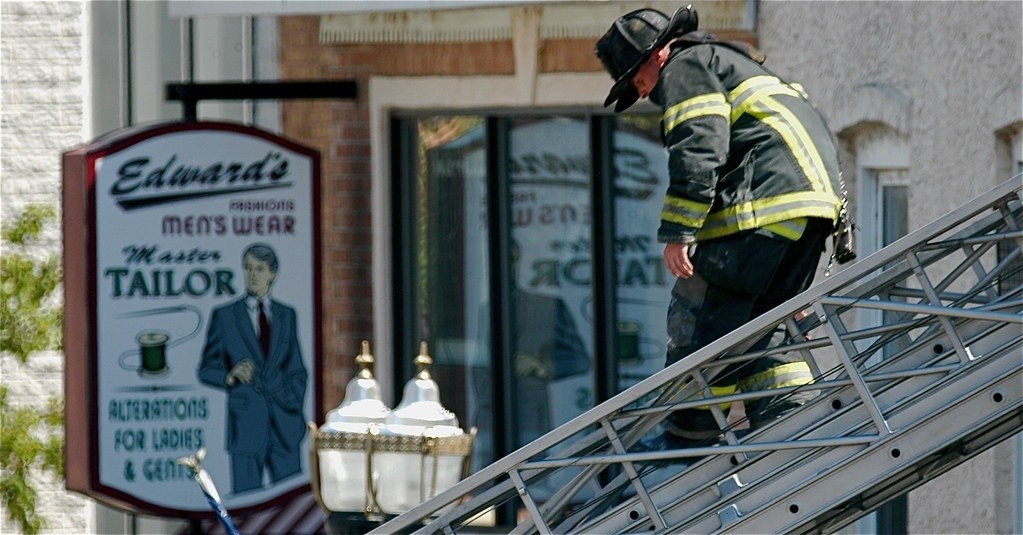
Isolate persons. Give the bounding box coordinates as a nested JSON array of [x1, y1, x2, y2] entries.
[[593, 6, 853, 464]]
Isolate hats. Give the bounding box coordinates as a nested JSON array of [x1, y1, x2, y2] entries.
[[593, 3, 699, 113]]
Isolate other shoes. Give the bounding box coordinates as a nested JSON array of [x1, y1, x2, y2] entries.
[[626, 432, 720, 468]]
